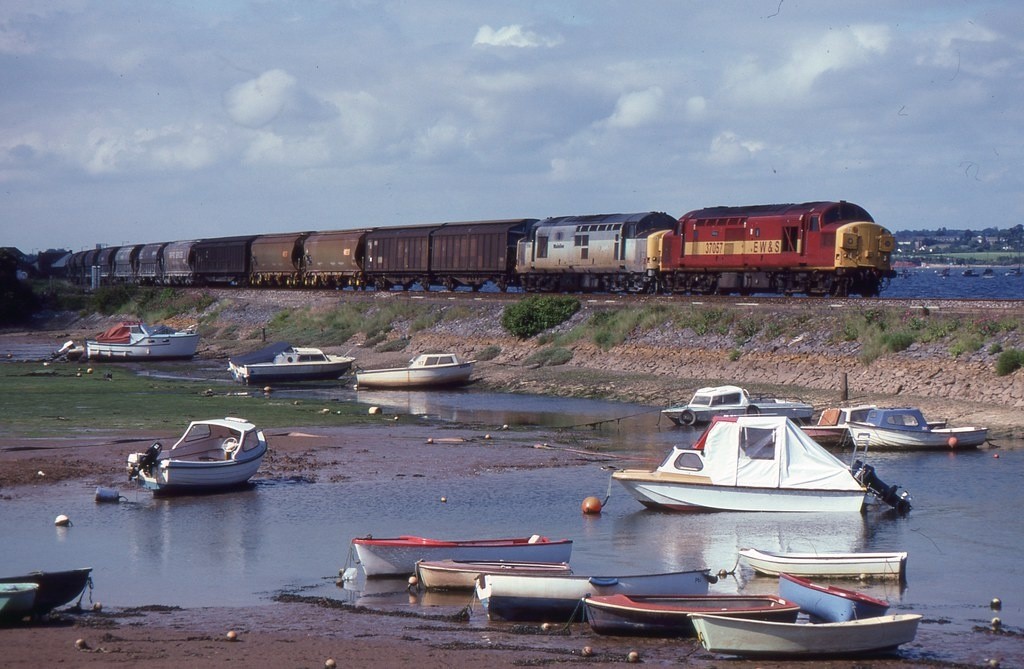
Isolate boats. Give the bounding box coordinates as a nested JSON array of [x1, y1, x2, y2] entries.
[[53, 322, 201, 362], [937, 268, 957, 276], [777, 573, 890, 623], [611, 412, 911, 513], [229, 344, 356, 386], [356, 353, 479, 388], [352, 534, 573, 580], [688, 613, 924, 657], [1005, 268, 1023, 277], [475, 567, 719, 622], [846, 406, 990, 451], [739, 547, 908, 580], [661, 385, 814, 427], [982, 269, 996, 279], [125, 417, 270, 498], [415, 559, 572, 595], [961, 269, 980, 277], [797, 400, 948, 446], [580, 593, 801, 637]]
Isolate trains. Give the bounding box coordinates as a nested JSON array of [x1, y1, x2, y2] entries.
[[68, 200, 899, 299]]
